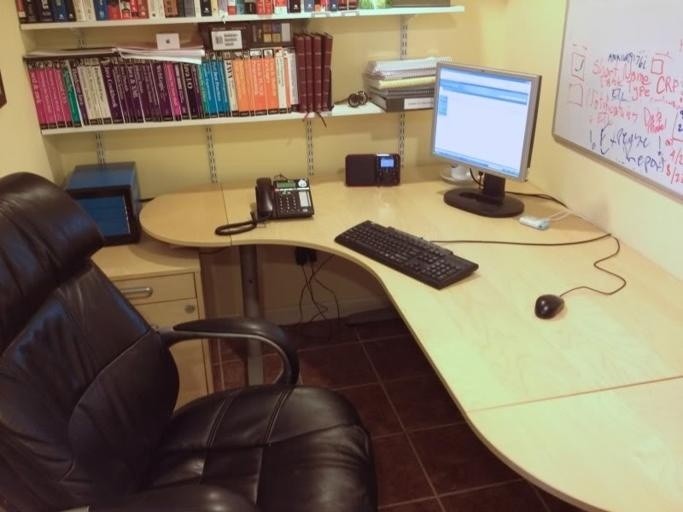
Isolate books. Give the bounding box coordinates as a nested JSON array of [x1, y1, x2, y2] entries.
[[23, 20, 334, 133], [365, 56, 451, 96], [16, 1, 458, 24]]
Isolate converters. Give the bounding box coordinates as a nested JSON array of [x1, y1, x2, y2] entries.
[[519, 216, 550, 230]]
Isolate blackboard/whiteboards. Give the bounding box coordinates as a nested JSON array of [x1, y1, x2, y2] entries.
[[552, 0, 683, 204]]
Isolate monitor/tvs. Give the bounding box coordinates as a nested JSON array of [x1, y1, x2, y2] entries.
[[429, 61, 542, 219]]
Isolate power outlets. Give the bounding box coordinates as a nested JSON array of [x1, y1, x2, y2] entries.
[[297, 247, 317, 266]]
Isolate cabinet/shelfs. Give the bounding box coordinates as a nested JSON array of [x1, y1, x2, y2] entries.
[[88, 241, 215, 412], [20, 4, 466, 136]]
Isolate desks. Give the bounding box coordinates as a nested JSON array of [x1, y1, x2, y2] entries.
[[140, 162, 683, 512]]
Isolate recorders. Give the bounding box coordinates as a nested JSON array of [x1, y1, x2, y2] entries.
[[345, 152, 401, 187]]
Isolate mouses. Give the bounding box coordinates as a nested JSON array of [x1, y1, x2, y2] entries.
[[534, 293, 565, 318]]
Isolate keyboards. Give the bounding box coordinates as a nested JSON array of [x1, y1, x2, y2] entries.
[[336, 219, 478, 290]]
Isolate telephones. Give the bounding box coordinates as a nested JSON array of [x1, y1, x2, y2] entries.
[[253, 177, 315, 224]]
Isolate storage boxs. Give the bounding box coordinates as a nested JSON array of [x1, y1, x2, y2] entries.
[[64, 161, 142, 244]]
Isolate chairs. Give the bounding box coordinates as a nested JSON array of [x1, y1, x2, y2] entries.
[[0, 173, 378, 512]]
